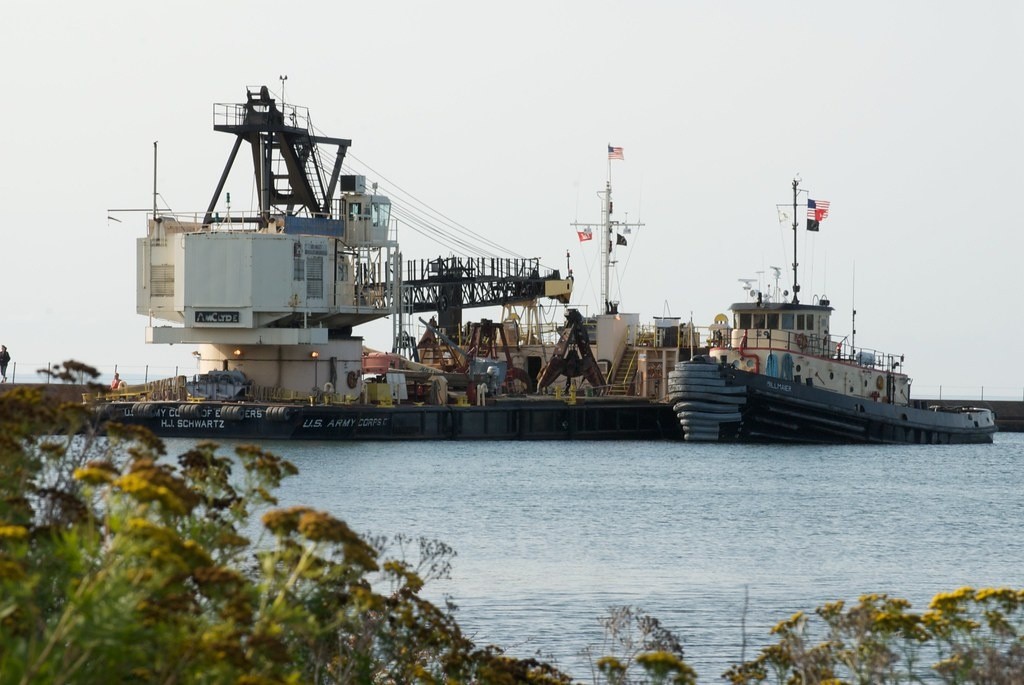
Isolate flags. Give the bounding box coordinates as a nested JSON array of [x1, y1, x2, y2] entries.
[[616, 233, 627, 246], [578, 231, 592, 242], [807, 198, 830, 231], [608, 145, 624, 161]]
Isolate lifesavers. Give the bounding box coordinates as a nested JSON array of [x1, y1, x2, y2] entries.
[[347, 370, 359, 390], [876, 374, 884, 391], [796, 332, 809, 349]]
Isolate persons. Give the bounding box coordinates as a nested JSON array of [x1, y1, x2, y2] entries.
[[0, 345, 11, 383], [110, 373, 124, 389]]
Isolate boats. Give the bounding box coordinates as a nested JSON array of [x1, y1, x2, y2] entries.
[[664, 172, 999, 444], [74, 87, 682, 442]]
